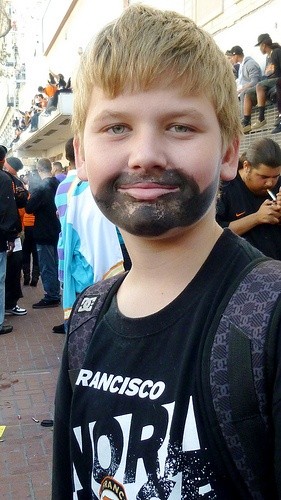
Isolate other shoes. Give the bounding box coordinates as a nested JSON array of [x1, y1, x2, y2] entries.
[[24, 275, 31, 286], [30, 277, 38, 286]]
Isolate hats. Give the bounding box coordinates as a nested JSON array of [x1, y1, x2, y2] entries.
[[254, 34, 269, 47], [5, 157, 23, 174], [227, 45, 243, 55]]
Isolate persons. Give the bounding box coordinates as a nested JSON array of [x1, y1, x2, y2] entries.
[[223, 45, 263, 101], [0, 144, 16, 334], [240, 33, 281, 134], [52, 4, 281, 500], [217, 136, 281, 260], [11, 157, 61, 309], [7, 72, 73, 150], [3, 157, 28, 316], [53, 138, 77, 335]]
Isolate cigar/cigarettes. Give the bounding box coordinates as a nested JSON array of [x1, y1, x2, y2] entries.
[[266, 188, 276, 201]]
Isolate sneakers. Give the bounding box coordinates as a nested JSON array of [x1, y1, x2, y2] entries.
[[251, 119, 267, 129], [52, 323, 65, 334], [270, 123, 281, 134], [273, 114, 281, 126], [5, 304, 27, 316], [32, 299, 59, 309], [0, 325, 13, 334], [242, 124, 252, 133]]
